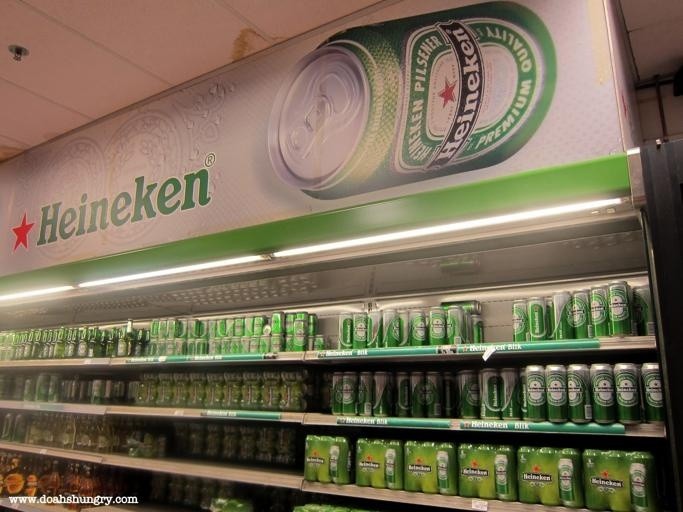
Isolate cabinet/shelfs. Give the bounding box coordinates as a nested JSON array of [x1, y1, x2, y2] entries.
[[0, 330, 679, 511]]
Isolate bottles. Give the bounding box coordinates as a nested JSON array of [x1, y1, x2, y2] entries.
[[23, 409, 170, 456], [0, 317, 136, 359], [0, 450, 116, 510]]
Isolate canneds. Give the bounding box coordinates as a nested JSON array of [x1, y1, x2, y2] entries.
[[266, 0, 558, 198], [438, 256, 479, 274], [0, 277, 666, 512], [564, 231, 637, 250]]
